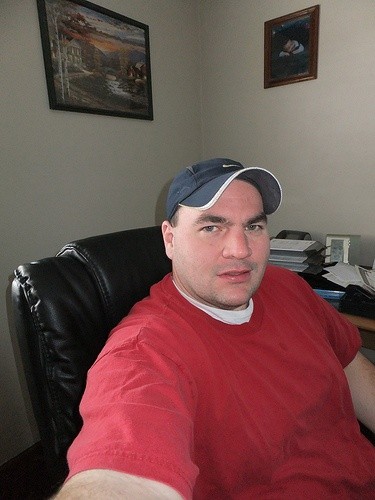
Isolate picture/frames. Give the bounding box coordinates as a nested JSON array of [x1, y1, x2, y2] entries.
[[36, 0, 154, 123], [263, 4, 320, 89]]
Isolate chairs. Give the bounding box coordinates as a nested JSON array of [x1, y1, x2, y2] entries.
[[10, 225, 173, 497]]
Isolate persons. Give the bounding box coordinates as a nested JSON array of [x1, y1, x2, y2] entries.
[[55, 157, 375, 500]]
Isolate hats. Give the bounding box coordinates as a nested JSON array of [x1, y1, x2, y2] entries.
[[164, 158, 282, 218]]
